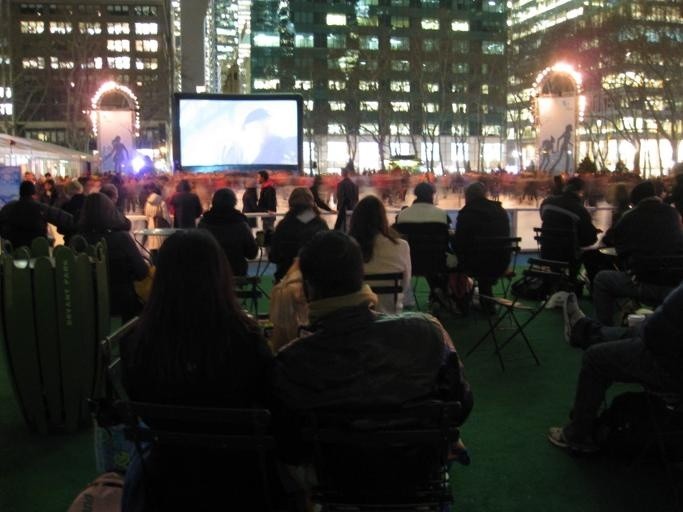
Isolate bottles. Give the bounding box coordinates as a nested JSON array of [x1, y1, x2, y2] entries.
[[257, 311, 272, 353]]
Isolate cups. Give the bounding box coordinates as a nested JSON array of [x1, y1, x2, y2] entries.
[[626, 314, 646, 329]]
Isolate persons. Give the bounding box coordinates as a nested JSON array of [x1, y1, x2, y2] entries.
[[546, 281, 681, 445], [537, 136, 554, 171], [1, 164, 682, 323], [137, 154, 158, 180], [103, 134, 128, 171], [274, 228, 474, 512], [94, 229, 275, 512], [551, 126, 571, 169]]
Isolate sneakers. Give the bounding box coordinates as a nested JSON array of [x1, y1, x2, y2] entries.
[[546, 424, 595, 454], [562, 292, 587, 344]]
